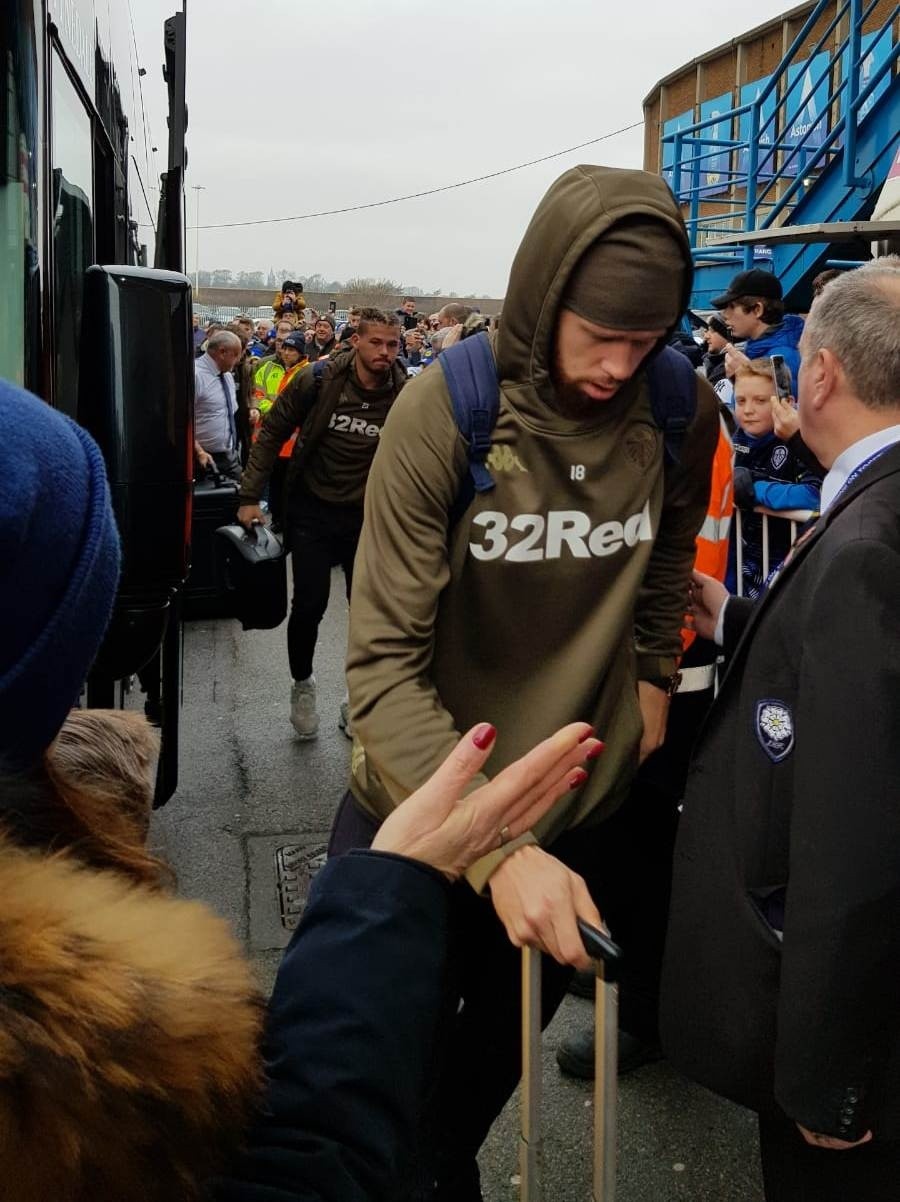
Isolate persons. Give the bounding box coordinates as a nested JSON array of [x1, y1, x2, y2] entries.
[[190, 296, 507, 533], [554, 270, 844, 1081], [272, 281, 306, 323], [192, 330, 242, 481], [330, 163, 722, 1202], [710, 269, 804, 401], [679, 256, 900, 1202], [205, 307, 414, 739], [0, 377, 608, 1202]]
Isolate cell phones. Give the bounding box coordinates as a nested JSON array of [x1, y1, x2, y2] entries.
[[578, 917, 631, 979], [770, 354, 790, 404]]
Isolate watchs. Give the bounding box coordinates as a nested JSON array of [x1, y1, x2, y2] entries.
[[808, 1130, 830, 1143], [642, 670, 684, 697]]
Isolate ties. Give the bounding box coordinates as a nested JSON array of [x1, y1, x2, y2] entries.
[[794, 487, 823, 542], [218, 372, 236, 454]]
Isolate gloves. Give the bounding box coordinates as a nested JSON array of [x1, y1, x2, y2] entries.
[[732, 465, 770, 510]]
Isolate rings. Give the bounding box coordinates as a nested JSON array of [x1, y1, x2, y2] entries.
[[500, 825, 512, 846]]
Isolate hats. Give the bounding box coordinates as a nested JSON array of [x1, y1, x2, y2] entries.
[[710, 311, 734, 340], [0, 379, 121, 798], [711, 269, 782, 310], [281, 330, 306, 354], [562, 216, 688, 329], [314, 314, 335, 332]]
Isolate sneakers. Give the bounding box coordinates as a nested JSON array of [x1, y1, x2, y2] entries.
[[289, 678, 321, 735], [338, 701, 353, 736]]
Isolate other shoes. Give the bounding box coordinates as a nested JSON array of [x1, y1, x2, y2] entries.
[[552, 1025, 664, 1079]]
